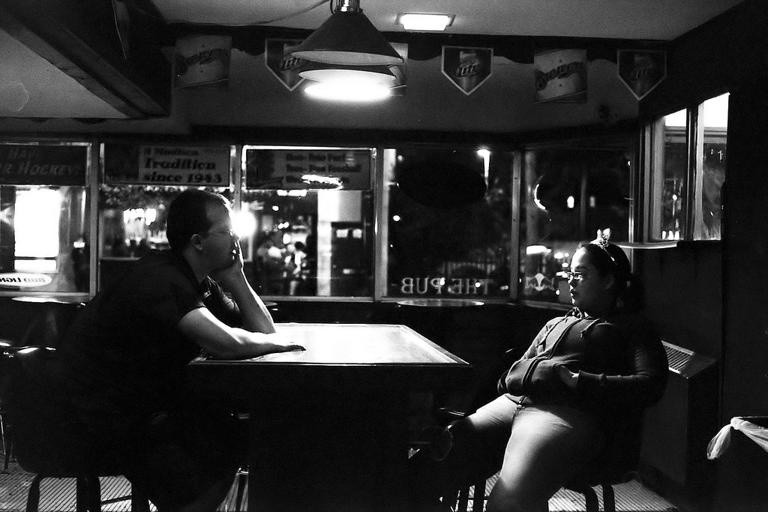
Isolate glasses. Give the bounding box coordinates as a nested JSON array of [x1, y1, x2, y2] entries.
[[198, 226, 235, 235], [563, 271, 608, 280]]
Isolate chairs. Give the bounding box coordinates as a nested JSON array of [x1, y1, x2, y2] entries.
[[7, 346, 148, 510]]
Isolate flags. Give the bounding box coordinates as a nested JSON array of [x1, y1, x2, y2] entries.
[[532, 46, 588, 107], [172, 32, 233, 93], [384, 41, 410, 99]]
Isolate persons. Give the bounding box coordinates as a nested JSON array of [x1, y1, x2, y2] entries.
[[384, 228, 670, 511], [60, 188, 306, 511]]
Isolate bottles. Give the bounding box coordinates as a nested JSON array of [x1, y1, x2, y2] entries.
[[439, 249, 507, 276]]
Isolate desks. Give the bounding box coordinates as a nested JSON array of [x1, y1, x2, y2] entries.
[[189, 322, 473, 510]]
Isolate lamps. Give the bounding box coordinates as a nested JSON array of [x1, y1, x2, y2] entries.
[[292, 4, 403, 106]]
